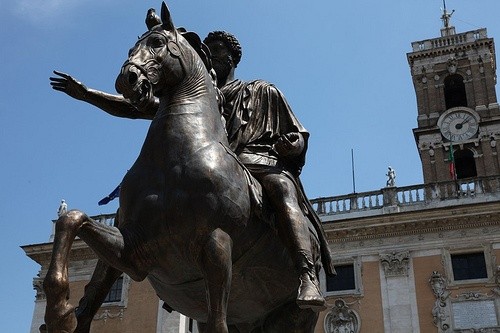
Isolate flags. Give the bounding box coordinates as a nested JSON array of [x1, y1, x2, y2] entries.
[[449, 133, 457, 180]]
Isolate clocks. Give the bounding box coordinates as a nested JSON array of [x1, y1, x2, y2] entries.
[[441, 112, 479, 142]]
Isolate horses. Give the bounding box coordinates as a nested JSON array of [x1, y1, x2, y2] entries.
[[38, 0, 322, 332]]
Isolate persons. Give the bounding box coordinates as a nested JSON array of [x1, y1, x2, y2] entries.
[[49, 31, 330, 313], [57, 199, 68, 217], [385, 166, 396, 187], [440, 9, 455, 28]]
